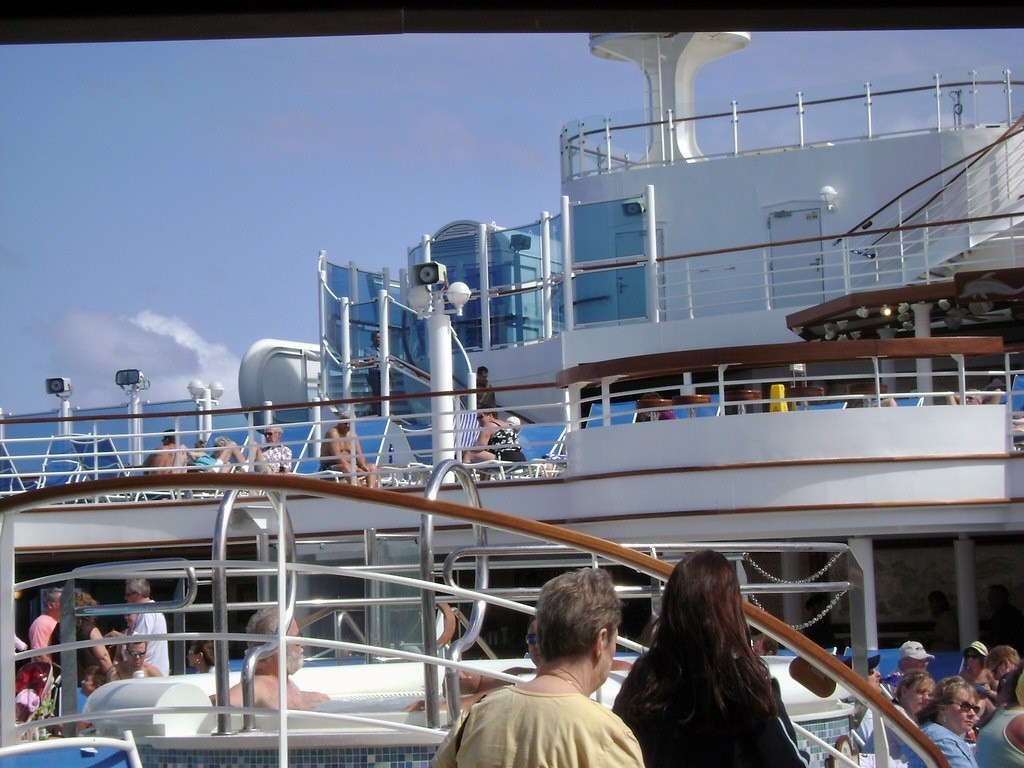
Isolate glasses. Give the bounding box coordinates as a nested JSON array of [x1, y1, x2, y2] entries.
[[949, 702, 981, 713], [963, 652, 981, 661], [526, 634, 538, 644], [476, 413, 487, 421], [868, 668, 877, 676], [263, 431, 277, 437], [127, 649, 147, 657], [991, 662, 1003, 677], [289, 632, 303, 646]]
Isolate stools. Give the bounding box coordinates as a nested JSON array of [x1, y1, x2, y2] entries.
[[636, 400, 672, 421], [726, 389, 760, 414], [784, 386, 825, 410], [671, 395, 711, 418], [846, 384, 888, 407]]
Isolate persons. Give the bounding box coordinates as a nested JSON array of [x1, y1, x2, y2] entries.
[[457, 403, 528, 480], [753, 632, 780, 657], [610, 549, 813, 768], [839, 384, 898, 409], [841, 640, 1024, 768], [475, 365, 499, 420], [403, 614, 634, 711], [945, 387, 1002, 405], [142, 427, 196, 501], [227, 603, 331, 714], [318, 412, 377, 489], [428, 569, 647, 768], [363, 330, 394, 416], [187, 640, 217, 674], [10, 574, 171, 739], [187, 422, 292, 495], [637, 391, 676, 423]]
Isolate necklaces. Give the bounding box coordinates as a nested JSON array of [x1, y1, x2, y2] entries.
[[541, 672, 583, 692]]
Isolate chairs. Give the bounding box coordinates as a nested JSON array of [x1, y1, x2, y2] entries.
[[0, 375, 1024, 504]]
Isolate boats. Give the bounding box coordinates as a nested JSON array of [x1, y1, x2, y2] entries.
[[0, 30, 1024, 767]]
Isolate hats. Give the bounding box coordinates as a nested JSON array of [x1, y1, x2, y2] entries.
[[968, 640, 987, 655], [505, 417, 521, 431], [898, 640, 934, 662], [845, 652, 880, 675]]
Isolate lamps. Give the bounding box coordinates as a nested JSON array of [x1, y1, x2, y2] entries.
[[903, 321, 914, 331], [838, 335, 848, 341], [938, 299, 950, 310], [880, 305, 891, 316], [850, 331, 861, 339], [856, 306, 869, 318], [824, 323, 834, 340], [836, 319, 848, 328], [899, 302, 909, 313], [821, 184, 839, 211]]
